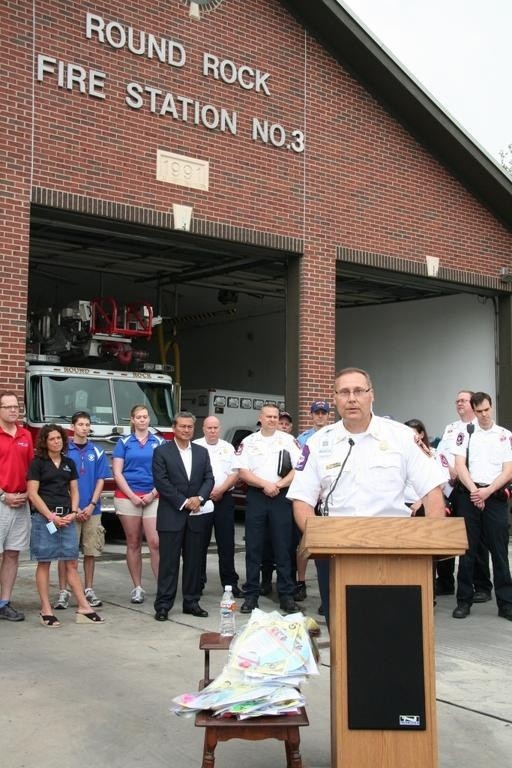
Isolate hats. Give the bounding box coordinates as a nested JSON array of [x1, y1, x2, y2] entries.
[[279, 412, 292, 421], [311, 401, 329, 412]]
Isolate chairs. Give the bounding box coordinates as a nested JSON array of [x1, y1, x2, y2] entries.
[[195, 632, 309, 767]]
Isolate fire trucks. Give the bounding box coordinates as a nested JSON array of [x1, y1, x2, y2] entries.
[[182, 388, 286, 511], [28, 299, 181, 513]]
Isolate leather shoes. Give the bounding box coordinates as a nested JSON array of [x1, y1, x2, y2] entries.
[[156, 609, 168, 621], [241, 598, 259, 614], [453, 607, 470, 619], [498, 608, 512, 621], [183, 606, 208, 617], [434, 586, 454, 594], [224, 586, 245, 598], [472, 592, 491, 602], [200, 591, 202, 595], [280, 600, 300, 613]]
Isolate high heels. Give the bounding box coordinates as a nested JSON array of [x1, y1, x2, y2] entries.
[[40, 613, 61, 628], [76, 612, 104, 624]]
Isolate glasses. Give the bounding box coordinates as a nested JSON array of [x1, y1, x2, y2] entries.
[[0, 406, 19, 408], [335, 388, 371, 396], [455, 399, 470, 403]]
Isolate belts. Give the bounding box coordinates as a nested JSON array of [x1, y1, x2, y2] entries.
[[35, 507, 68, 515]]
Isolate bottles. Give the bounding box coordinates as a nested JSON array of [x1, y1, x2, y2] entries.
[[220, 584, 237, 637]]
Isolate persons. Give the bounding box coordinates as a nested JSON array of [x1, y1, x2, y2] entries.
[[405, 391, 511, 620], [0, 391, 332, 628], [285, 368, 450, 627]]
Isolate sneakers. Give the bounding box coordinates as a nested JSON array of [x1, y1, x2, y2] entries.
[[54, 590, 71, 608], [131, 587, 146, 603], [242, 583, 247, 590], [294, 587, 308, 601], [318, 605, 324, 615], [259, 584, 272, 595], [1, 602, 24, 621], [84, 590, 102, 607]]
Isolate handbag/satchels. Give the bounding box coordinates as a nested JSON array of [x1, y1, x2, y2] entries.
[[448, 480, 480, 520]]
